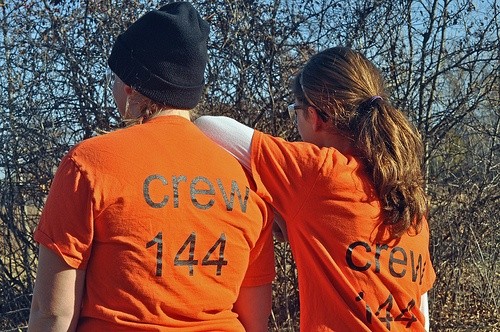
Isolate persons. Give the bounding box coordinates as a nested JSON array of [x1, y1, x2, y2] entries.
[[193, 45, 436, 332], [27, 1, 277, 332]]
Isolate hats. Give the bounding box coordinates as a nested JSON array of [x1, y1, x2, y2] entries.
[[108, 2, 210, 108]]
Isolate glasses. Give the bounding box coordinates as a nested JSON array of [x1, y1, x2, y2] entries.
[[288, 103, 330, 123]]
